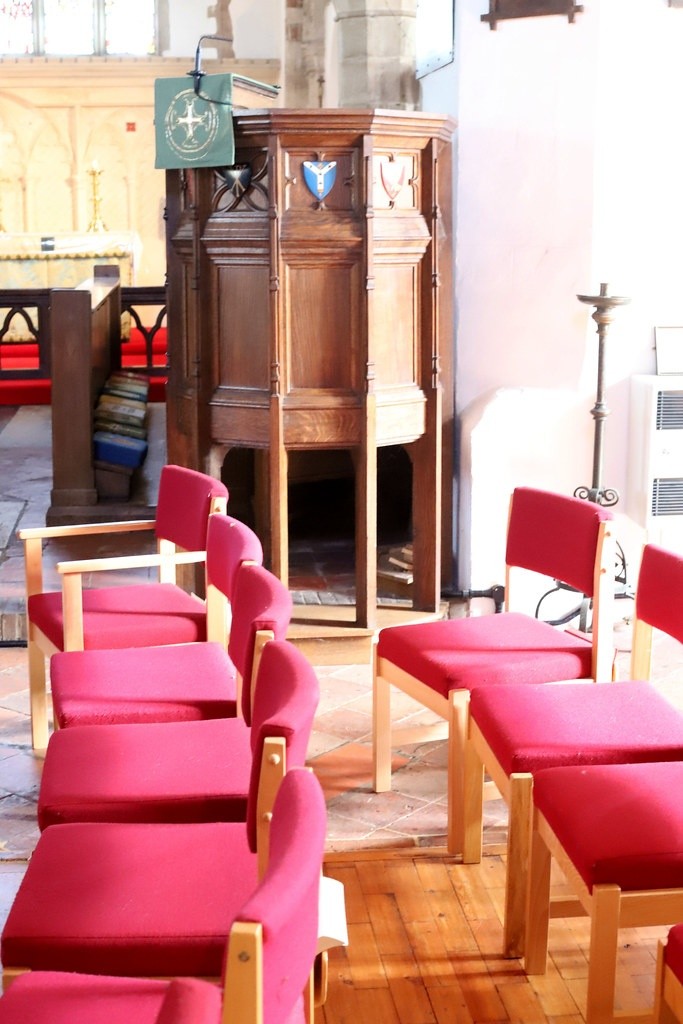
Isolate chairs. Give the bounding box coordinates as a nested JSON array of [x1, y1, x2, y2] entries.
[[0, 465, 683, 1024]]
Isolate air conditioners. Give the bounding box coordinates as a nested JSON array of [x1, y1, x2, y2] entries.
[[626, 371, 683, 560]]
